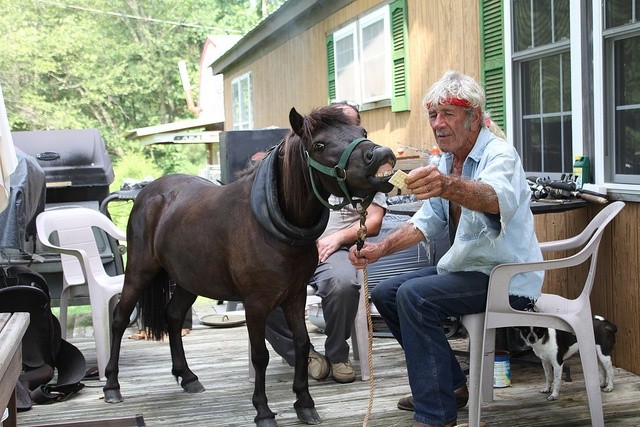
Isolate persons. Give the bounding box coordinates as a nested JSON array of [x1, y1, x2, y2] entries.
[[350, 70, 545, 427], [251, 102, 390, 383]]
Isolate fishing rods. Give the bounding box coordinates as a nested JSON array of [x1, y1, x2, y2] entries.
[[397, 141, 609, 204]]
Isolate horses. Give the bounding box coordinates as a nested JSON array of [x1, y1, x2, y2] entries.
[[104, 103, 396, 427]]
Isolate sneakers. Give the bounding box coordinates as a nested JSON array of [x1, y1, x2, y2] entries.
[[306, 345, 328, 380], [329, 360, 356, 380]]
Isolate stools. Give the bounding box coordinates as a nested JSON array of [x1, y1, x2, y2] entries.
[[0, 310, 31, 422]]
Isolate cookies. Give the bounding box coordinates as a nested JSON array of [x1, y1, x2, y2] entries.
[[388, 170, 409, 191]]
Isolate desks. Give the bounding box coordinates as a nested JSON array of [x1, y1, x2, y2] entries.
[[386, 197, 587, 376]]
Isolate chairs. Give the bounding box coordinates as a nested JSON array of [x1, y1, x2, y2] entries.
[[460, 201, 626, 427], [36, 205, 127, 382], [249, 268, 371, 385]]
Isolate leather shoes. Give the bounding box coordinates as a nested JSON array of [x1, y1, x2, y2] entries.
[[407, 421, 459, 427], [397, 387, 470, 411]]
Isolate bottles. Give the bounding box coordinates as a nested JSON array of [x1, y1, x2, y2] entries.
[[493, 351, 511, 388]]
